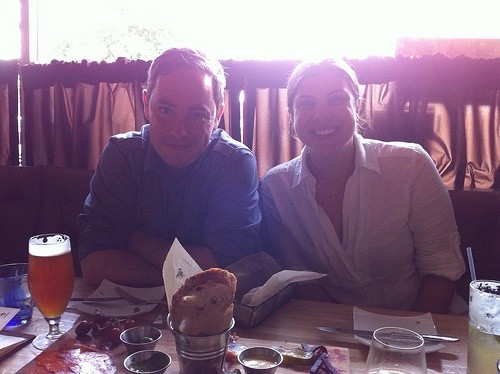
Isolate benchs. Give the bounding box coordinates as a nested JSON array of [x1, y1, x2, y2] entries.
[[0, 167, 500, 315]]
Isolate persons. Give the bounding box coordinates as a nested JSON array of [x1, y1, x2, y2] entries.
[[261, 57, 469, 316], [76, 47, 262, 288]]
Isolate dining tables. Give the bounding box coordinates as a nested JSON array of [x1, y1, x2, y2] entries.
[[0, 278, 470, 374]]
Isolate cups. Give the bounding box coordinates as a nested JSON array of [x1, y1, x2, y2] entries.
[[167, 311, 235, 374], [0, 263, 33, 326], [365, 327, 427, 374], [467, 279, 500, 374]]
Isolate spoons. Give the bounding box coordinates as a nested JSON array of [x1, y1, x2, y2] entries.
[[114, 286, 167, 306]]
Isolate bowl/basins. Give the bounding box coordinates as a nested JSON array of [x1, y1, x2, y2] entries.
[[120, 326, 162, 352], [237, 346, 283, 374], [124, 350, 172, 374]]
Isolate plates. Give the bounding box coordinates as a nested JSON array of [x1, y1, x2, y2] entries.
[[84, 279, 166, 316], [352, 306, 446, 354]]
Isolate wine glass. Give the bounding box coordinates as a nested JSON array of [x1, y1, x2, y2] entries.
[[28, 233, 75, 350]]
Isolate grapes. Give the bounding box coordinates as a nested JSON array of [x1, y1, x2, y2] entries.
[[75, 311, 137, 346]]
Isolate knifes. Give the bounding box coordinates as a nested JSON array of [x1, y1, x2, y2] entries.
[[69, 296, 125, 302], [315, 326, 460, 343]]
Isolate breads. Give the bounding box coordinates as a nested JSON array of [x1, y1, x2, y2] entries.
[[171, 268, 237, 336]]
